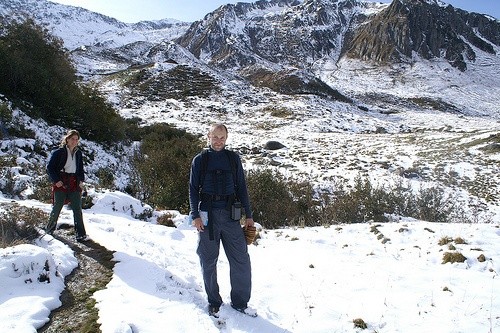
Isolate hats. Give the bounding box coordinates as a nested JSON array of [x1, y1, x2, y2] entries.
[[243, 227, 256, 244]]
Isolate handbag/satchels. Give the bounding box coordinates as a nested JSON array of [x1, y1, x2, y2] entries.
[[232, 201, 242, 220]]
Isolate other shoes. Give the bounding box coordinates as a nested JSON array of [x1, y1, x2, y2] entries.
[[45, 231, 52, 234], [76, 236, 86, 241], [231, 302, 257, 318], [205, 309, 219, 318]]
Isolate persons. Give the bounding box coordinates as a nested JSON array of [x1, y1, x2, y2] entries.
[[188, 123, 259, 329], [45, 130, 89, 242]]
